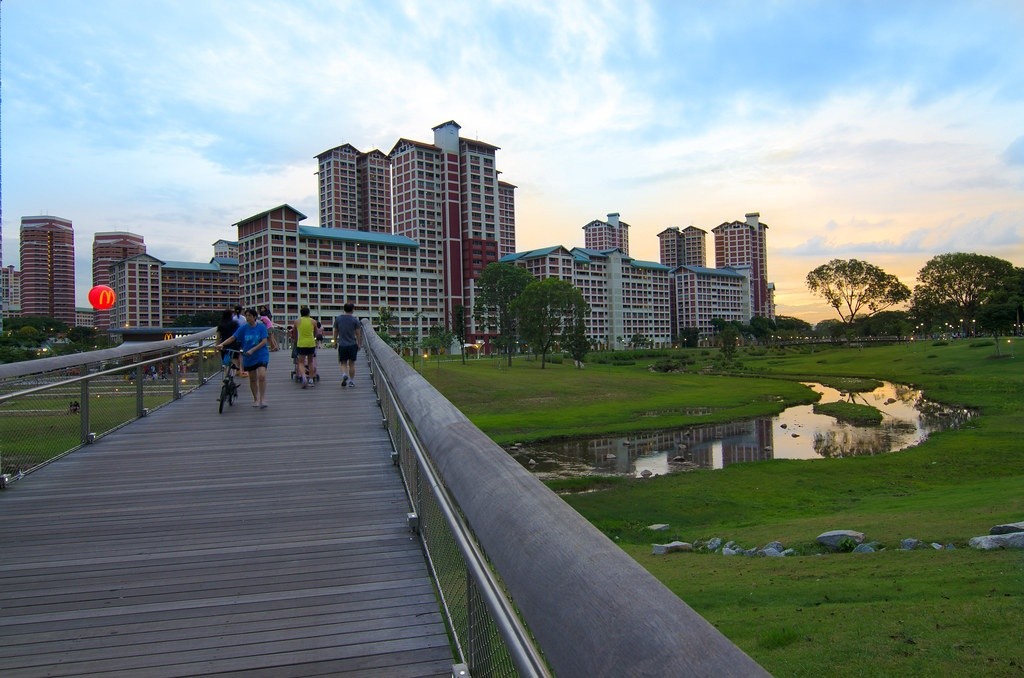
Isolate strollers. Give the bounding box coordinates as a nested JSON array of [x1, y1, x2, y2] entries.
[[291, 341, 320, 382]]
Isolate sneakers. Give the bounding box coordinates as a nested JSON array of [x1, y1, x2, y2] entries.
[[340, 373, 348, 386], [259, 401, 268, 408], [348, 380, 355, 387], [252, 401, 260, 407], [308, 379, 314, 387], [301, 380, 307, 388]]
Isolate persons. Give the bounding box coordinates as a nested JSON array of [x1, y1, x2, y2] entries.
[[214, 303, 325, 375], [215, 309, 272, 408], [73, 401, 80, 410], [127, 362, 190, 381], [949, 329, 977, 339], [332, 302, 362, 388], [291, 307, 320, 389], [69, 401, 75, 410]]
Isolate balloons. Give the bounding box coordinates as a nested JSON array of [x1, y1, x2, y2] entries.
[[88, 285, 116, 309]]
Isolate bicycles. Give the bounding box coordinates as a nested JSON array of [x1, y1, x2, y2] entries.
[[216, 348, 247, 414]]
